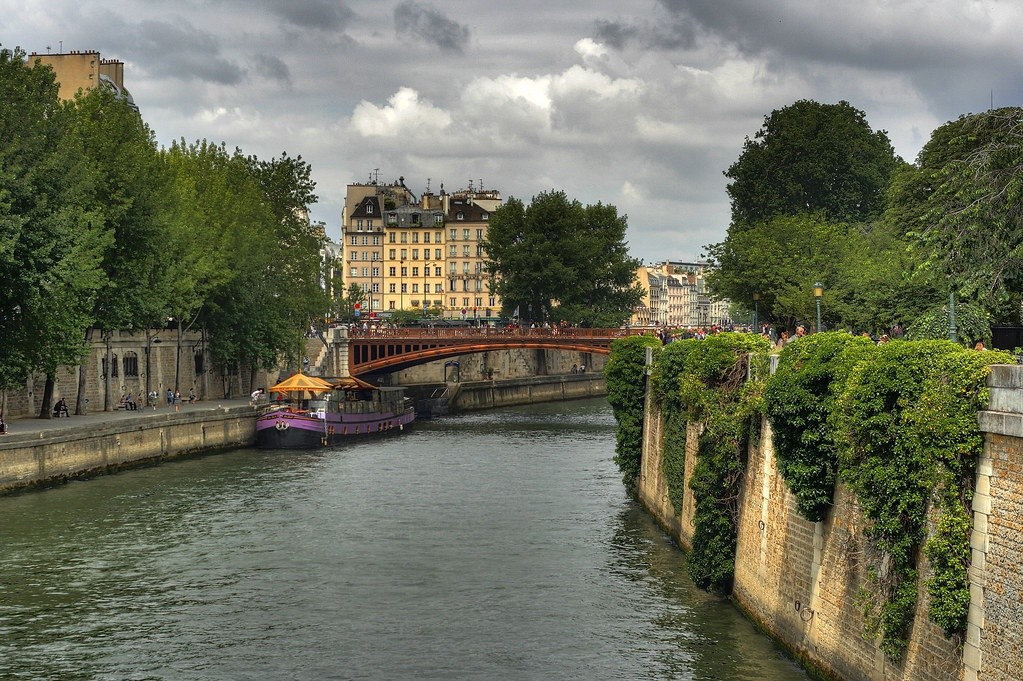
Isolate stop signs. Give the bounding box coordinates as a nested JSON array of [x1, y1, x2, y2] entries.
[[370, 313, 374, 317], [461, 310, 466, 315], [354, 304, 360, 309]]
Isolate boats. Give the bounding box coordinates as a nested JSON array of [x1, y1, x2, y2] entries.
[[254, 370, 416, 449]]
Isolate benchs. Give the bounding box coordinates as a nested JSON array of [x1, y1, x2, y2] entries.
[[52, 410, 66, 417], [117, 402, 131, 410], [180, 397, 190, 403]]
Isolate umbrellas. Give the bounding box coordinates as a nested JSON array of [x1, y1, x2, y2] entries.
[[267, 368, 331, 411]]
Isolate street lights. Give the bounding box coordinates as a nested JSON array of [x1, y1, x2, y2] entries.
[[752, 290, 760, 333], [704, 306, 706, 327], [424, 260, 436, 308], [812, 281, 825, 332], [695, 305, 701, 330], [368, 258, 382, 313], [367, 289, 371, 329], [399, 261, 404, 312]]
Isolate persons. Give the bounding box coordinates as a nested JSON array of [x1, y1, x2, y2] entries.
[[703, 323, 734, 336], [167, 388, 173, 407], [188, 388, 197, 404], [465, 323, 523, 336], [352, 322, 399, 337], [420, 321, 435, 337], [529, 319, 570, 336], [656, 323, 705, 345], [136, 389, 146, 413], [303, 355, 310, 371], [248, 389, 262, 405], [172, 389, 181, 412], [120, 395, 130, 410], [151, 389, 159, 410], [126, 392, 137, 410], [54, 398, 70, 417], [746, 322, 804, 348]]
